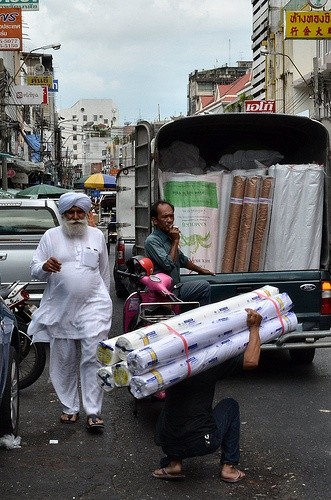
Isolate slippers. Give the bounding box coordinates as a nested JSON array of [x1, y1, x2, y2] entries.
[[221, 467, 247, 482], [61, 411, 80, 423], [86, 414, 104, 428], [153, 467, 186, 478]]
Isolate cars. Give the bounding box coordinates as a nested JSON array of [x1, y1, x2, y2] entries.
[[0, 198, 63, 310], [0, 296, 24, 443]]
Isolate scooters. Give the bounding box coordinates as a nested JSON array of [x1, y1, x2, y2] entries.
[[115, 255, 200, 423]]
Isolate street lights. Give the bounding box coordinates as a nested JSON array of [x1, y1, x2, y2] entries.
[[259, 49, 320, 120], [54, 118, 85, 190], [0, 40, 62, 192]]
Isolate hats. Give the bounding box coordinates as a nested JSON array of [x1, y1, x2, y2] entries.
[[58, 191, 91, 214]]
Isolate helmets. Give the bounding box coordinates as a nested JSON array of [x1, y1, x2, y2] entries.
[[126, 255, 154, 288]]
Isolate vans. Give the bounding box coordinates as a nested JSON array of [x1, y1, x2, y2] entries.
[[114, 111, 330, 372]]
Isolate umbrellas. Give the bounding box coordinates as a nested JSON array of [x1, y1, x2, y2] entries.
[[18, 183, 67, 195], [75, 172, 118, 188]]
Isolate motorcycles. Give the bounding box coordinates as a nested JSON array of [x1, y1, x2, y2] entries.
[[0, 280, 46, 391]]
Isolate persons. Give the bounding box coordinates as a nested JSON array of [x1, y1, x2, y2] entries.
[[150, 309, 264, 481], [27, 193, 113, 428], [144, 200, 216, 305]]
